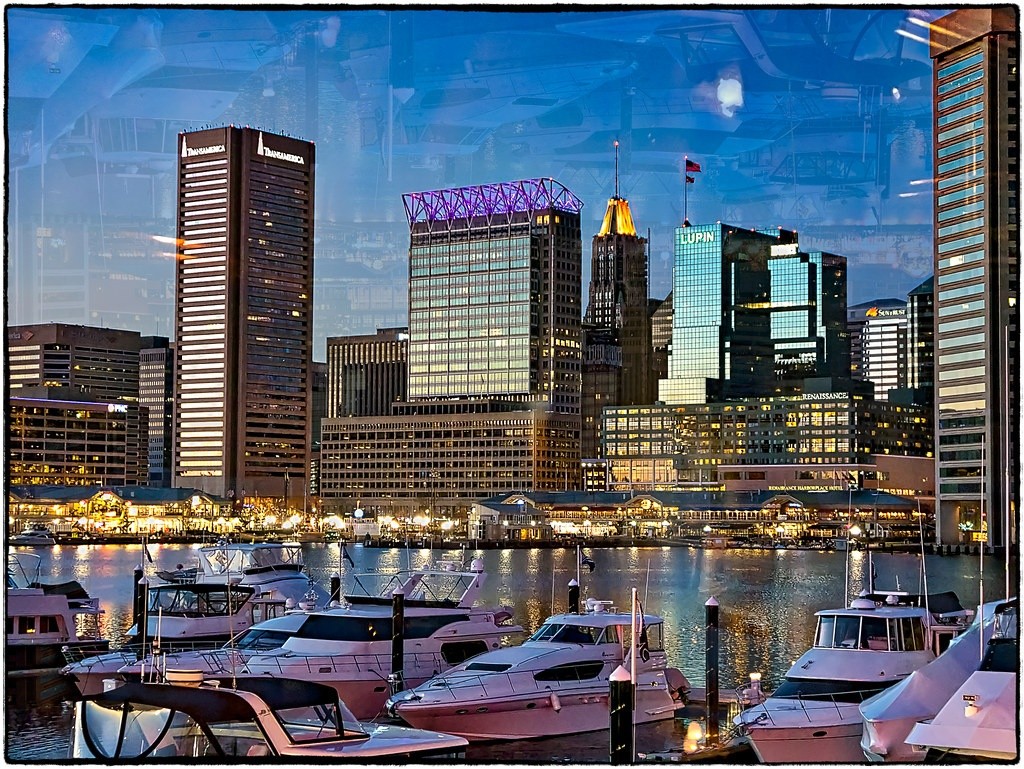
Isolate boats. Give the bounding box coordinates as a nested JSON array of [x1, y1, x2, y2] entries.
[[215, 540, 228, 547], [56, 542, 690, 767], [9, 525, 59, 545], [731, 485, 1016, 764], [6, 553, 111, 678], [739, 542, 826, 550], [282, 542, 302, 548]]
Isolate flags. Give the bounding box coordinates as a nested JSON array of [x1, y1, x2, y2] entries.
[[686, 161, 700, 172], [580, 552, 595, 573], [686, 176, 694, 183], [638, 602, 650, 663], [144, 546, 152, 563], [343, 546, 354, 567]]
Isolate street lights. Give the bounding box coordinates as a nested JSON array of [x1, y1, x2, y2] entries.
[[775, 526, 783, 541], [80, 500, 85, 531], [703, 525, 712, 539], [583, 520, 591, 536], [429, 469, 440, 527], [631, 521, 636, 535], [503, 520, 508, 539], [126, 501, 131, 532], [662, 521, 670, 538], [531, 521, 536, 539]]
